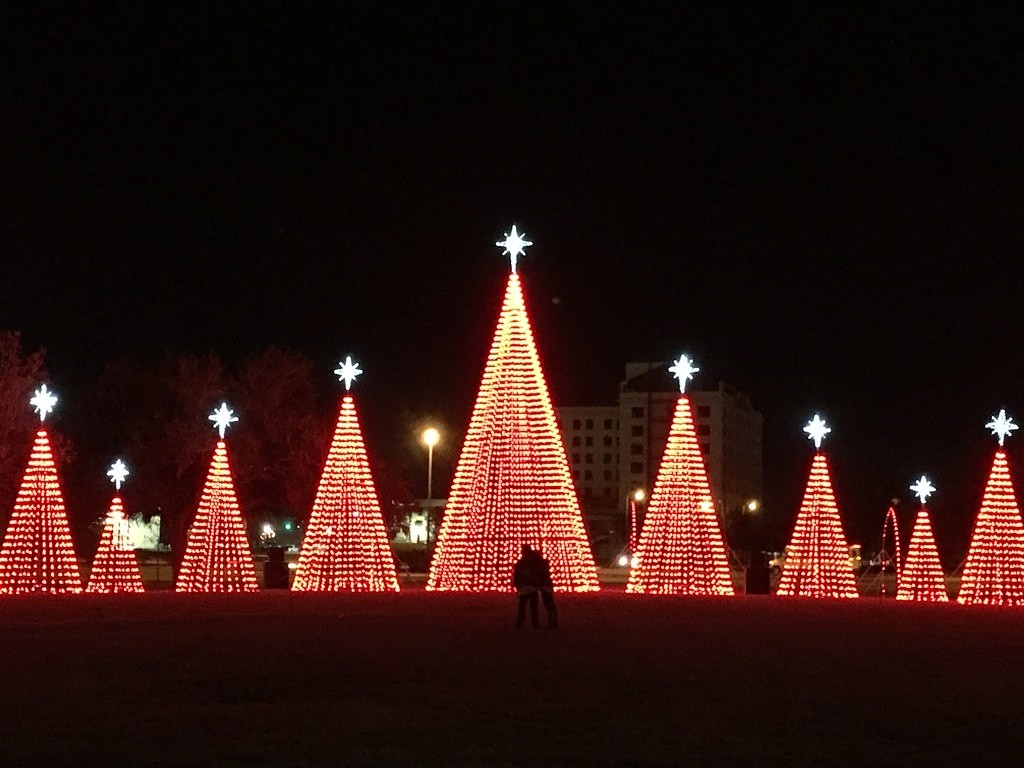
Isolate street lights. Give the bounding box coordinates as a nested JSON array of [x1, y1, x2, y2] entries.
[[625, 488, 646, 532], [422, 426, 442, 499]]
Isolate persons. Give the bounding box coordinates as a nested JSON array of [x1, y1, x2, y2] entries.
[[513, 545, 557, 628]]
